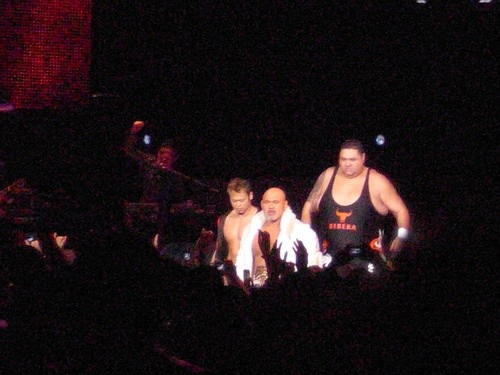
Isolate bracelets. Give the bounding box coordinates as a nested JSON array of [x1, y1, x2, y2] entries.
[[398, 228, 409, 238]]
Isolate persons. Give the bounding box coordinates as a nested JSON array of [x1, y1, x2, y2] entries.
[[0, 120, 500, 375]]
